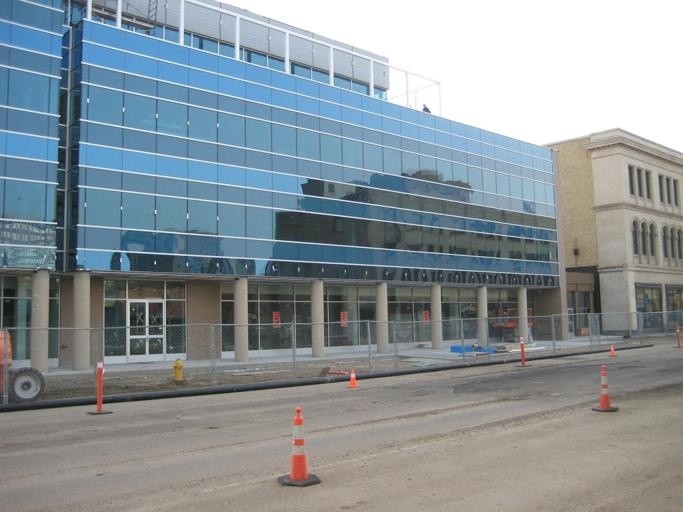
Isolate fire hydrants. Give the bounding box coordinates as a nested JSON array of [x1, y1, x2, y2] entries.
[[173, 359, 183, 381]]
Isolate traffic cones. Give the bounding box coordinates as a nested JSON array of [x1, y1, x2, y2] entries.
[[516, 335, 531, 367], [591, 365, 617, 412], [87, 360, 114, 415], [345, 369, 360, 388], [277, 407, 321, 487], [607, 343, 615, 357], [673, 323, 681, 348]]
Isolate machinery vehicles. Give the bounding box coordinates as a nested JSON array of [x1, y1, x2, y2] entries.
[[0, 329, 44, 406]]
